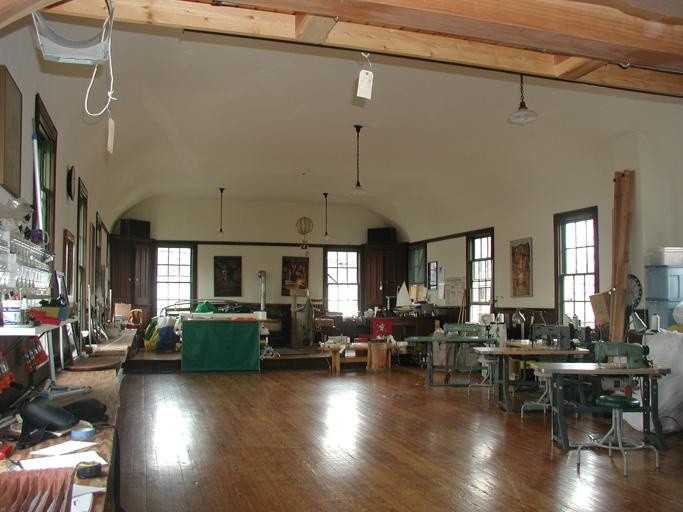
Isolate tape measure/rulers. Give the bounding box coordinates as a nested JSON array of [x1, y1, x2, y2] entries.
[[77, 462, 102, 478]]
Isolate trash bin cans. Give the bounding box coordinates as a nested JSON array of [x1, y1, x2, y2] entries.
[[370, 339, 387, 369]]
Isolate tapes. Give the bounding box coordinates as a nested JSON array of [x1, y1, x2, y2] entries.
[[71, 427, 96, 438]]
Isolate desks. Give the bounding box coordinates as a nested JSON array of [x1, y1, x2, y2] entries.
[[313, 310, 672, 479]]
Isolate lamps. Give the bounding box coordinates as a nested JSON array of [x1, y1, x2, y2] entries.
[[347, 124, 370, 198], [507, 73, 537, 126], [210, 189, 333, 241]]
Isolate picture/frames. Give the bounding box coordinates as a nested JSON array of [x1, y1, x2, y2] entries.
[[508, 237, 534, 299], [427, 260, 439, 290]]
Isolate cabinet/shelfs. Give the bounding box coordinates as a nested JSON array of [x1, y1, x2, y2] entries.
[[181, 316, 262, 374], [0, 200, 139, 512]]
